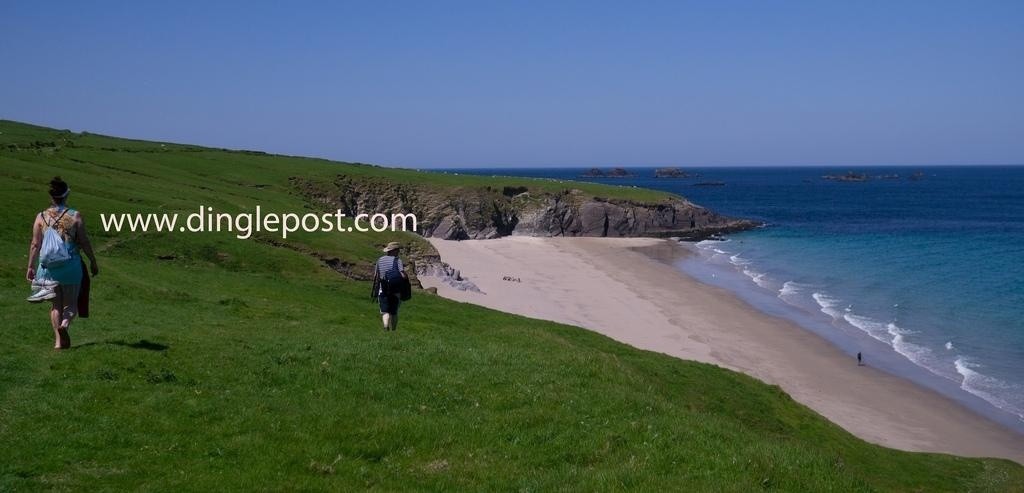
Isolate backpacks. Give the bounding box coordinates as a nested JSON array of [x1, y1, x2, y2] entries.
[[40, 209, 72, 270]]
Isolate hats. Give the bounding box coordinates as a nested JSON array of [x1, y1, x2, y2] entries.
[[383, 242, 403, 253]]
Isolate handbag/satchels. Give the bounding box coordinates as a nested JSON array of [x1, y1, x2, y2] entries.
[[386, 270, 411, 301]]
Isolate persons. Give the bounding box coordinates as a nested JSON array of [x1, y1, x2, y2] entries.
[[856, 351, 862, 365], [370, 241, 405, 332], [25, 175, 99, 350]]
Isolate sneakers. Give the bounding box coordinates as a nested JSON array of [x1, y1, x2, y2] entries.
[[54, 325, 71, 349], [383, 326, 395, 331], [28, 279, 60, 301]]
